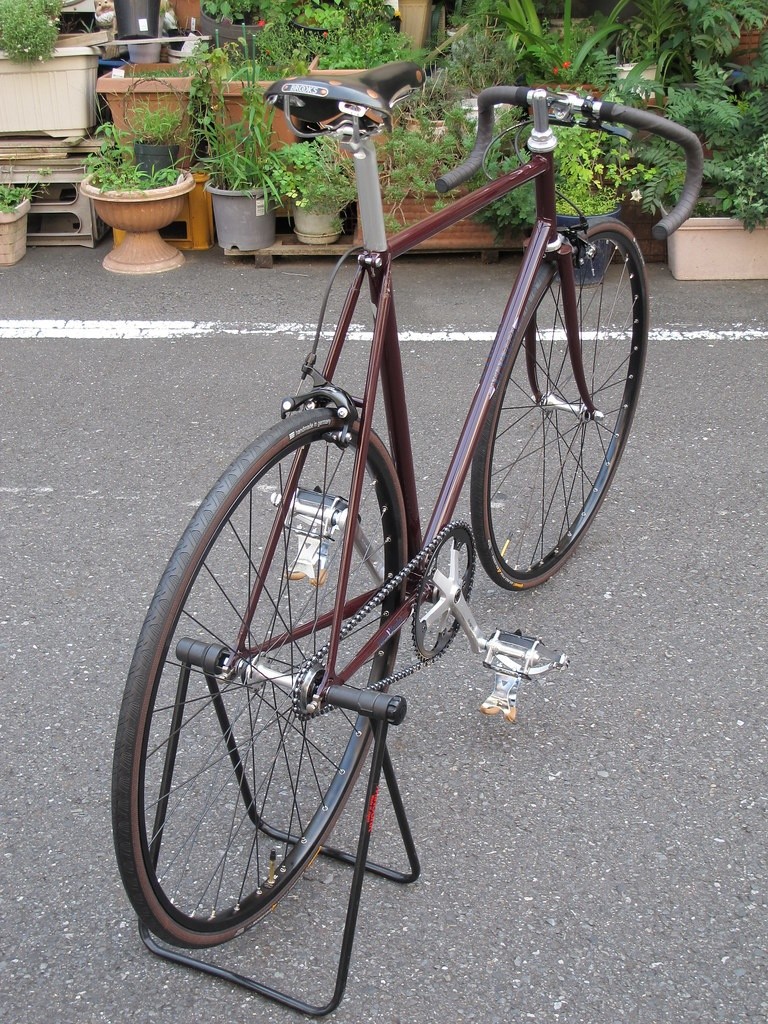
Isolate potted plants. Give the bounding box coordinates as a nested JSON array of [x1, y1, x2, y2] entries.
[[554, 187, 620, 290], [353, 138, 537, 249], [96, 62, 195, 169], [0, 167, 52, 266], [81, 122, 196, 275], [306, 20, 431, 77], [719, 0, 762, 65], [199, 0, 278, 59], [403, 68, 451, 143], [452, 47, 512, 134], [186, 65, 275, 252], [0, 0, 101, 138], [613, 24, 657, 99], [289, 0, 402, 61], [642, 60, 768, 281], [208, 24, 299, 156], [273, 135, 355, 235]]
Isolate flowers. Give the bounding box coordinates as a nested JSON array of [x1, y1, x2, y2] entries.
[[546, 60, 574, 84]]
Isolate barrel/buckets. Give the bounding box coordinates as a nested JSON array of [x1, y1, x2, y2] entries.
[[127, 17, 164, 63], [113, 0, 161, 40], [166, 45, 192, 64]]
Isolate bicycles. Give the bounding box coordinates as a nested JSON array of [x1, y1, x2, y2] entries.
[[110, 61, 704, 1017]]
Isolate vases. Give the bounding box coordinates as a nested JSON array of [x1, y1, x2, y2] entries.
[[528, 82, 608, 116]]
